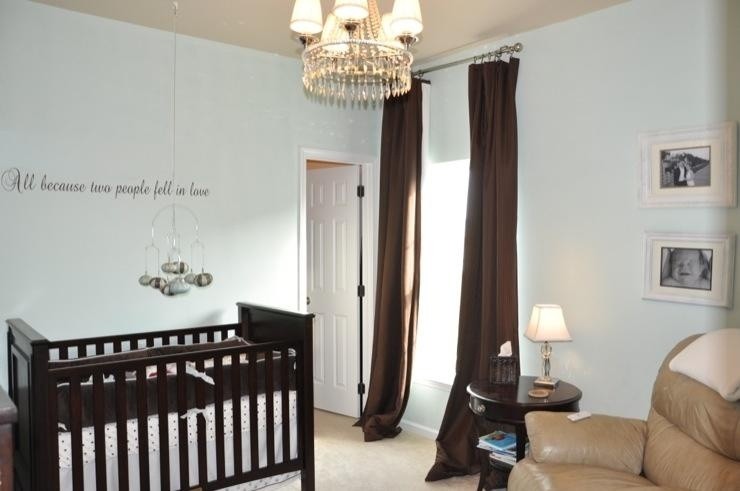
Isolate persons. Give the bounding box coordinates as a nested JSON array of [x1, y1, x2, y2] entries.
[[661, 248, 710, 290], [682, 162, 695, 186], [674, 161, 687, 186]]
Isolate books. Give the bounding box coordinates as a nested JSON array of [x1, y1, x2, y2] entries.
[[476, 430, 529, 466]]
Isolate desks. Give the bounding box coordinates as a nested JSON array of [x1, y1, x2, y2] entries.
[[466, 375, 583, 491]]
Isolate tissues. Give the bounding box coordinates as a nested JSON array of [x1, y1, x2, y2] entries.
[[490, 341, 517, 386]]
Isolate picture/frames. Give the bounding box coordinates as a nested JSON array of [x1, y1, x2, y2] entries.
[[641, 228, 738, 313], [638, 124, 738, 212]]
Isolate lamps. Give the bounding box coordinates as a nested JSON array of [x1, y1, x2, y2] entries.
[[138, 0, 217, 300], [284, 0, 427, 105], [522, 302, 574, 392]]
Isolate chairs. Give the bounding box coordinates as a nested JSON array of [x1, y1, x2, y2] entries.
[[504, 333, 740, 491]]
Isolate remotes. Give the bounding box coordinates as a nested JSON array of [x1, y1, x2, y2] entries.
[[567, 411, 592, 422]]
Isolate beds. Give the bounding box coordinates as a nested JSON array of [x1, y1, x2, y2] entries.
[[6, 302, 316, 490]]
